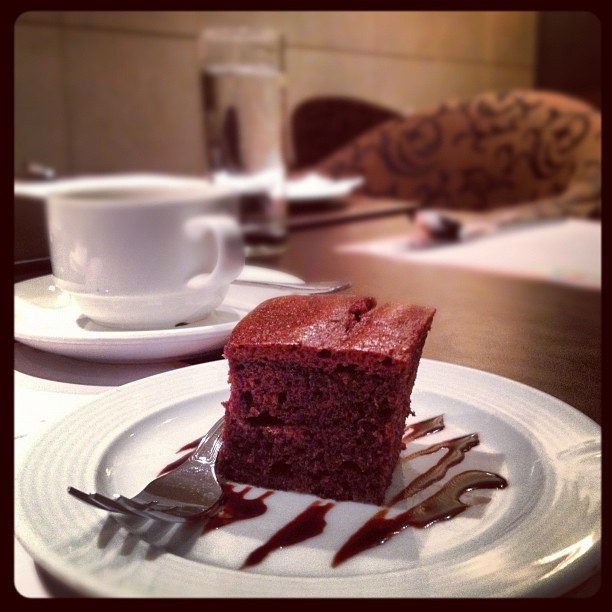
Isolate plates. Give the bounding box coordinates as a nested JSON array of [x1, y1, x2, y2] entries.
[[14, 266, 307, 362], [15, 357, 602, 600]]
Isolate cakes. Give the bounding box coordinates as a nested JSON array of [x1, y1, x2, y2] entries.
[[215, 293, 437, 505]]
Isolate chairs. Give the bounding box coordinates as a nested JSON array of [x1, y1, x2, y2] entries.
[[301, 87, 602, 226]]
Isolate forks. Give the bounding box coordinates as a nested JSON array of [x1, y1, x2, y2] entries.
[[66, 417, 225, 523]]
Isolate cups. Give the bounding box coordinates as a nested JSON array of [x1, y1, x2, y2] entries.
[[45, 176, 245, 327], [198, 26, 289, 256]]
